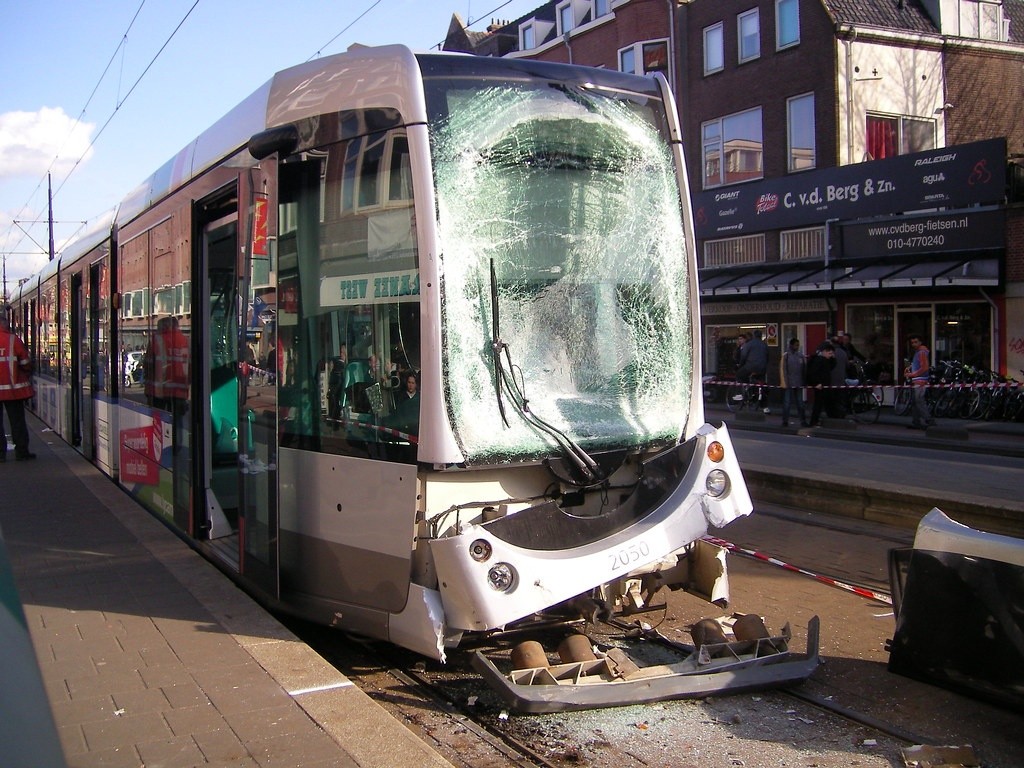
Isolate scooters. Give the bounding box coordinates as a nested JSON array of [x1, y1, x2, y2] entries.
[[124, 360, 142, 387]]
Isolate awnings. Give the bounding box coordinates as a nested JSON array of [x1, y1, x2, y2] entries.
[[698, 254, 1000, 296]]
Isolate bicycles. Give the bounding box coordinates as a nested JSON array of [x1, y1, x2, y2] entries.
[[844, 358, 885, 425], [893, 359, 1024, 423], [725, 364, 764, 412]]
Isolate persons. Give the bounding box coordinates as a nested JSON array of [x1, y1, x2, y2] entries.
[[0, 305, 38, 462], [731, 328, 871, 428], [121, 316, 419, 458], [903, 334, 937, 429]]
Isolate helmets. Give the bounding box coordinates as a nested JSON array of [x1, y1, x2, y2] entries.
[[0, 305, 8, 319]]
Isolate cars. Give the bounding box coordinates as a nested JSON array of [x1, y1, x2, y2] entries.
[[127, 351, 143, 365]]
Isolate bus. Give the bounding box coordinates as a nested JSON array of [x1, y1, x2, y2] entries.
[[0, 42, 823, 714]]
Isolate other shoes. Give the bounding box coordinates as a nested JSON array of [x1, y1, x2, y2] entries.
[[14, 452, 37, 460], [762, 407, 771, 414], [906, 423, 922, 429], [0, 458, 5, 462], [800, 421, 808, 426], [732, 394, 743, 401]]
